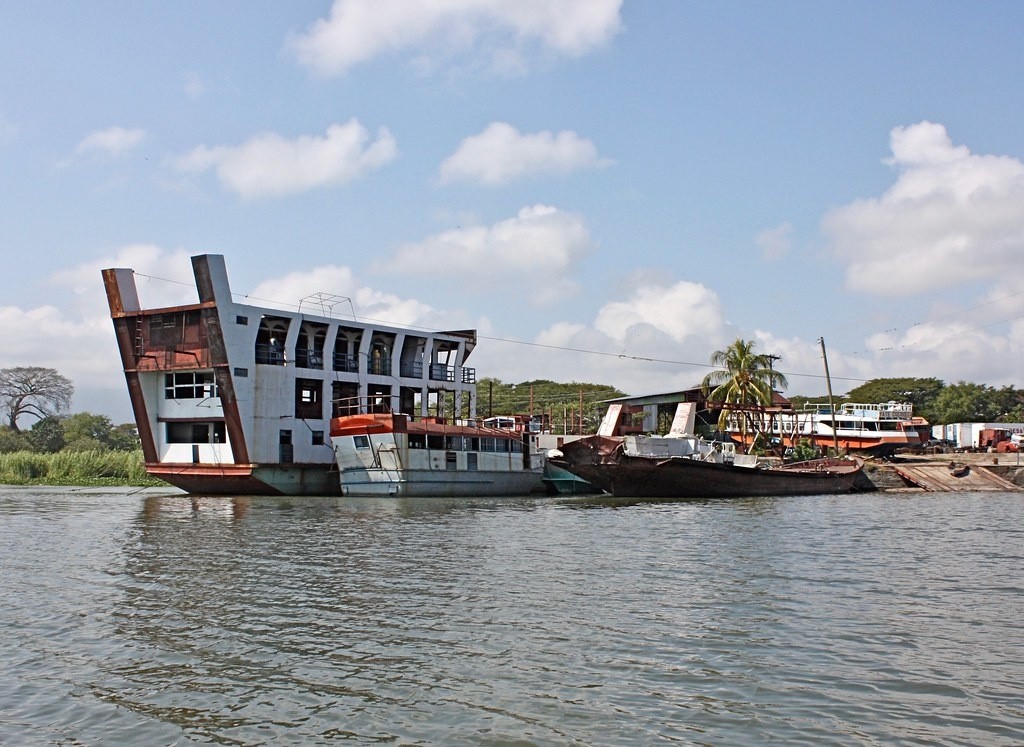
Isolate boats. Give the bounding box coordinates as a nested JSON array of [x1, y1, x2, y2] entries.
[[540, 455, 608, 495], [329, 394, 542, 497], [101, 254, 476, 496], [722, 400, 931, 458], [545, 402, 865, 497]]
